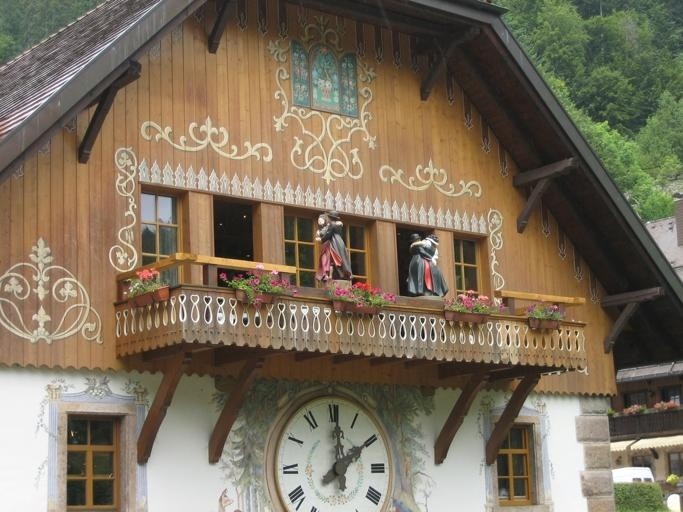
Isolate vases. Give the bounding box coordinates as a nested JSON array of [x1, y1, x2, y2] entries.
[[235, 287, 275, 304], [132, 285, 169, 307], [445, 310, 489, 323], [331, 299, 384, 314]]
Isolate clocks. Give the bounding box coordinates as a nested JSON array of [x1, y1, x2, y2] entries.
[[263, 385, 397, 512]]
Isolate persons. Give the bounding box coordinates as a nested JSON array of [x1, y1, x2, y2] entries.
[[317, 212, 343, 280], [406, 234, 448, 300], [316, 209, 353, 280], [409, 233, 428, 299]]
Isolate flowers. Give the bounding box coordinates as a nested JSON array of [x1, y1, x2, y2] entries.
[[324, 281, 397, 308], [220, 263, 299, 308], [123, 268, 160, 297], [444, 290, 504, 315]]
